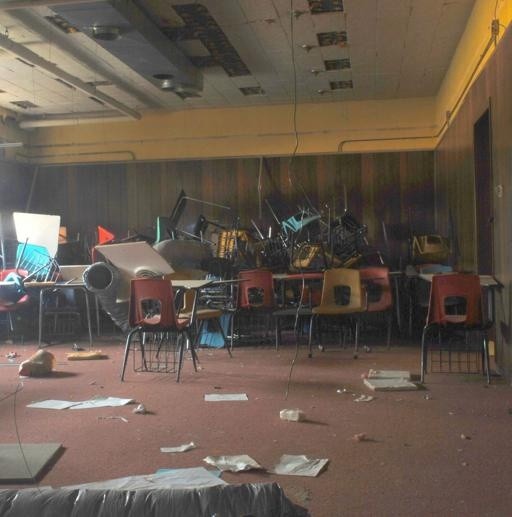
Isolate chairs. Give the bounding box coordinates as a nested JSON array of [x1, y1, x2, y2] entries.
[[231, 270, 280, 351], [1, 180, 500, 346], [419, 273, 492, 386], [175, 279, 234, 369], [120, 278, 198, 385], [356, 266, 392, 348], [306, 268, 365, 360]]
